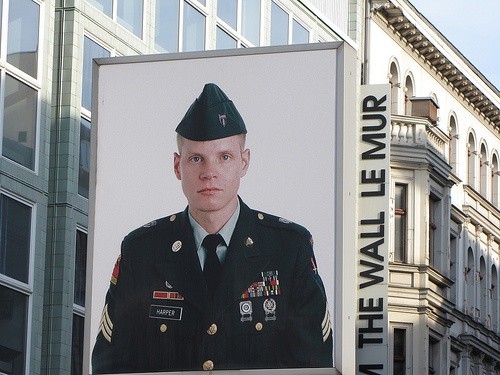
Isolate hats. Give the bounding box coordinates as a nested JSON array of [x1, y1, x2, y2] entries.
[[175, 83, 247, 141]]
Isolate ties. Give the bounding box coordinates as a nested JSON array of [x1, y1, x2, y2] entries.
[[200, 234, 223, 291]]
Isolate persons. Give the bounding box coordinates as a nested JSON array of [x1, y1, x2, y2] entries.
[[90, 83, 335, 375]]
